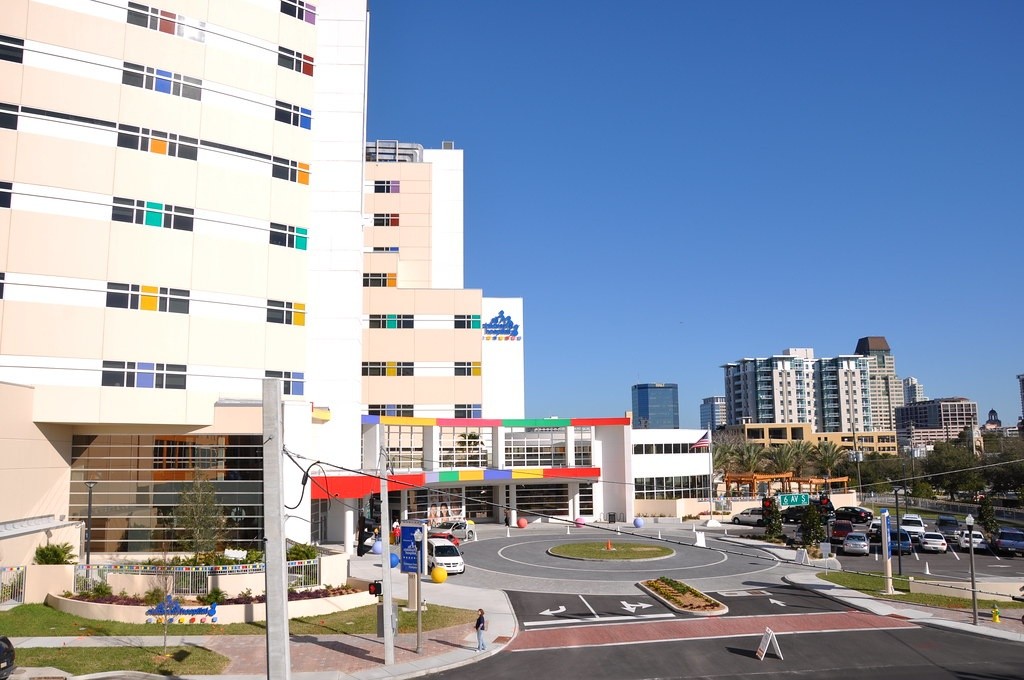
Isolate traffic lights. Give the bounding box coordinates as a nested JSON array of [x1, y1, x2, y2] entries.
[[357, 516, 374, 557], [368, 582, 381, 595]]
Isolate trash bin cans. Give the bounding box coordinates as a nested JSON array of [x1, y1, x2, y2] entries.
[[608, 512, 616, 523]]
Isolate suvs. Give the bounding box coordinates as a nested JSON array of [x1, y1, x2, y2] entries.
[[865, 521, 882, 546], [779, 498, 834, 525]]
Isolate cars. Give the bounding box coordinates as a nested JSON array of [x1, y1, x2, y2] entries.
[[890, 528, 913, 555], [428, 520, 476, 542], [732, 507, 766, 527], [899, 513, 928, 542], [834, 506, 874, 523], [431, 534, 459, 547], [918, 531, 948, 553], [841, 532, 873, 556], [993, 526, 1024, 557], [956, 530, 988, 551], [0, 633, 18, 680]]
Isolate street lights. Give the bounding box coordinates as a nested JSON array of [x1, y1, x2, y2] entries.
[[81, 481, 96, 591], [965, 514, 981, 626]]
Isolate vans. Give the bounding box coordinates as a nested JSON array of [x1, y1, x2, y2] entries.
[[830, 519, 853, 544], [427, 537, 466, 575], [933, 516, 963, 542]]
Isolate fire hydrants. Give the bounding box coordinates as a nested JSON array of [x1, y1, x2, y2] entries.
[[991, 603, 1001, 623]]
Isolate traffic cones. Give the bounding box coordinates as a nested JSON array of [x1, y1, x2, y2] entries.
[[473, 532, 478, 541], [657, 530, 662, 540], [616, 526, 621, 536], [506, 526, 511, 538], [724, 528, 729, 538], [923, 561, 931, 575], [691, 523, 696, 533], [605, 539, 612, 551], [565, 524, 570, 534]]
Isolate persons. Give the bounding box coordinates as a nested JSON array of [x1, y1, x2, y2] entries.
[[440, 503, 449, 523], [392, 519, 400, 545], [504, 508, 510, 527], [428, 505, 441, 531], [450, 503, 462, 523], [473, 609, 487, 652]]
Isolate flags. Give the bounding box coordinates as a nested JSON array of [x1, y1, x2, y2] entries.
[[688, 432, 709, 450]]
